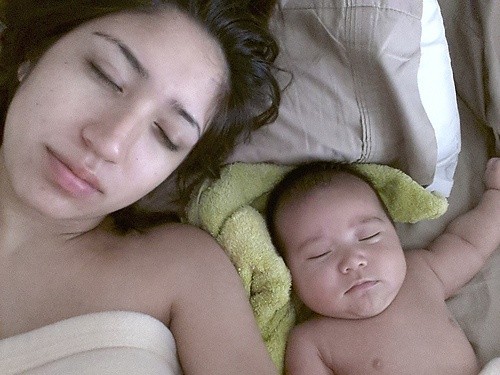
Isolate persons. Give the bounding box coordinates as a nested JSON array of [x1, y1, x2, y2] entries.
[[266, 154, 500, 374], [0, 0, 293, 375]]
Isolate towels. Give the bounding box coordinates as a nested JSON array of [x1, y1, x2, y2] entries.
[[180, 163, 449, 374]]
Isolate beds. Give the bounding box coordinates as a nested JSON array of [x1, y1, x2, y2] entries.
[[136, 0, 500, 375]]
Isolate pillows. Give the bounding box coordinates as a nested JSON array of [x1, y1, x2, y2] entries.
[[137, 0, 464, 215]]
